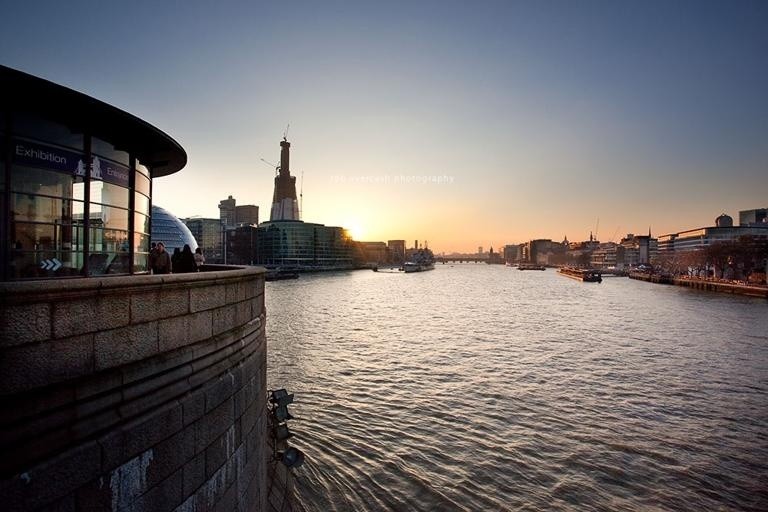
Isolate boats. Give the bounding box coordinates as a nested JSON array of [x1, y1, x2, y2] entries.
[[557, 265, 603, 284], [626, 269, 673, 284], [516, 262, 546, 271], [402, 245, 436, 274]]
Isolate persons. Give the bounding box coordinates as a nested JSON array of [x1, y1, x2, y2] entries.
[[171, 247, 182, 270], [152, 242, 171, 274], [175, 244, 198, 272], [193, 247, 205, 268], [151, 242, 156, 252]]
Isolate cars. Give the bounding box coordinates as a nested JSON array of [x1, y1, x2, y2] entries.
[[263, 263, 300, 270], [679, 274, 745, 286]]
